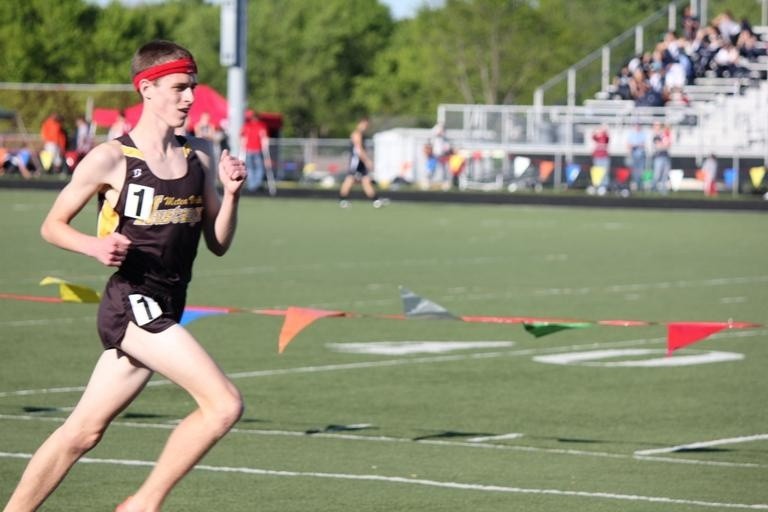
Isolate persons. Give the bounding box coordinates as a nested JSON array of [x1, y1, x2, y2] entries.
[[1, 40, 248, 512]]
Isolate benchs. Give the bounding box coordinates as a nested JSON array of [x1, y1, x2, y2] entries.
[[556, 27, 766, 149]]
